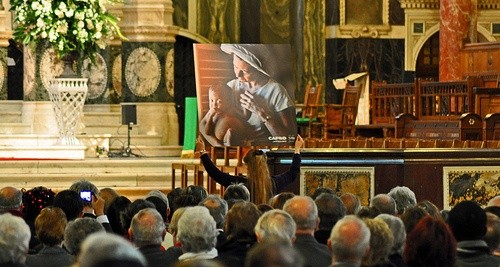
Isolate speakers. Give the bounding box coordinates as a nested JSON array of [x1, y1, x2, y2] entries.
[[121, 105, 137, 125]]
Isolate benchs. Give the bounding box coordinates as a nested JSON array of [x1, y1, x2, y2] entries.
[[359, 116, 402, 138]]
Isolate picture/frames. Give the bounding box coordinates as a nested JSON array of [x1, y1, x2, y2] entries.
[[300, 167, 375, 209], [443, 166, 500, 210]]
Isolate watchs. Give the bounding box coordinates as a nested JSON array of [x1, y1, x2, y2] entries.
[[200, 150, 206, 155]]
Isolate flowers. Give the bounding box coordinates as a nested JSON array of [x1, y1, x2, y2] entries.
[[8, 0, 130, 70]]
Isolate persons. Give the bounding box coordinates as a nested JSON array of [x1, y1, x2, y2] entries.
[[200, 45, 296, 146], [0, 178, 500, 267], [196, 134, 304, 204]]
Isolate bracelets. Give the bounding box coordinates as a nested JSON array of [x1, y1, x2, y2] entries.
[[265, 115, 272, 121]]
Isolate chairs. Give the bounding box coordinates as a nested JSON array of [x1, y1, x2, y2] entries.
[[292, 79, 363, 139]]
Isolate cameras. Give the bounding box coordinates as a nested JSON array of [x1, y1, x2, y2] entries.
[[80, 191, 95, 203]]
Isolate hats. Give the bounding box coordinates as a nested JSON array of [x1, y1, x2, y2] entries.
[[220, 43, 275, 78]]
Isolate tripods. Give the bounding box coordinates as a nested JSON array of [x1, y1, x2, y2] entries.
[[120, 124, 139, 158]]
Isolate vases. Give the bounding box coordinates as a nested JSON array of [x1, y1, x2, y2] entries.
[[55, 57, 81, 78]]
